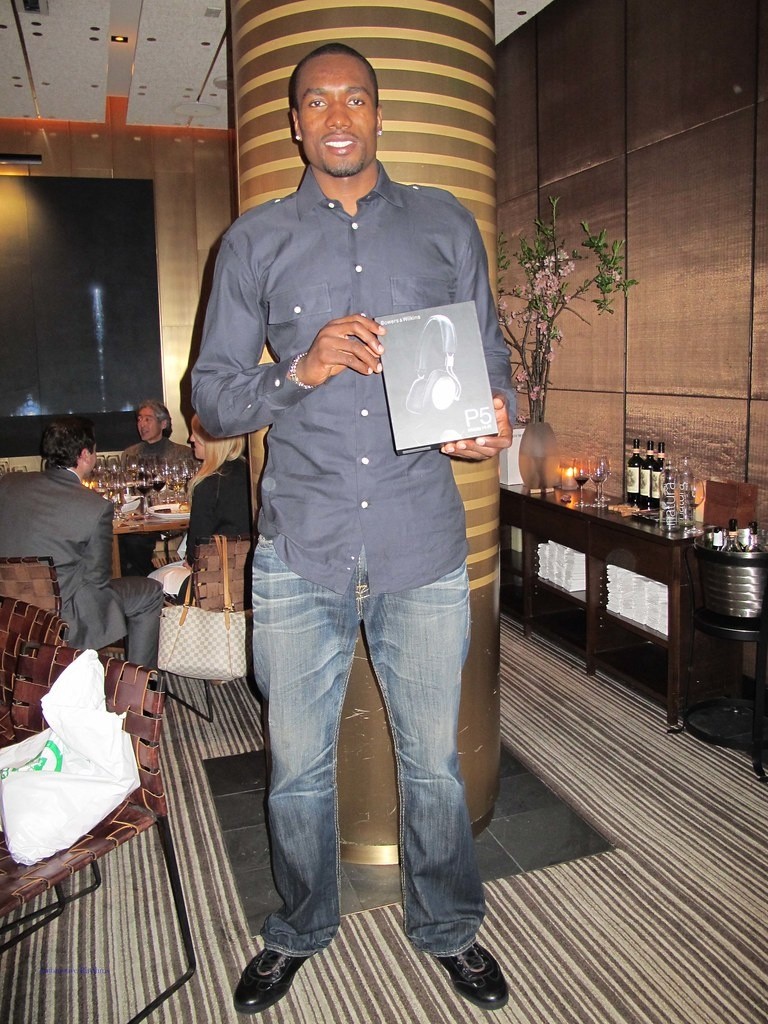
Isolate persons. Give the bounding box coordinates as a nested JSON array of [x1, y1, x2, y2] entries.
[[0, 415, 164, 673], [160, 412, 252, 605], [192, 42, 516, 1013], [120, 400, 195, 571]]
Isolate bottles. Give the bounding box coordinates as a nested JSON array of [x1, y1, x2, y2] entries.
[[702, 518, 768, 552], [625, 439, 695, 533]]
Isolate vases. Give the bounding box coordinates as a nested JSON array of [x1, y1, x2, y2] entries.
[[519, 421, 563, 491]]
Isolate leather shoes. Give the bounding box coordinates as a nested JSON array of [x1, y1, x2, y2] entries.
[[233, 947, 311, 1013], [434, 941, 510, 1010]]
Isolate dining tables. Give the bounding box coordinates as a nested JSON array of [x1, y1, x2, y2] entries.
[[111, 506, 191, 581]]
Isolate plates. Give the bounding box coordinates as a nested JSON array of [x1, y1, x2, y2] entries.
[[147, 504, 190, 521]]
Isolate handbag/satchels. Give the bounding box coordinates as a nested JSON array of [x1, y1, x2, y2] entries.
[[0, 646, 143, 866], [158, 536, 253, 686]]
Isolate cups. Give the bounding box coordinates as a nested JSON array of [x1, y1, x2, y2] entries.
[[0, 457, 28, 478]]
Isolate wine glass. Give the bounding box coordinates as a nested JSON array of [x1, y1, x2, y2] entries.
[[573, 458, 591, 508], [595, 456, 610, 502], [590, 457, 608, 508], [81, 455, 202, 518]]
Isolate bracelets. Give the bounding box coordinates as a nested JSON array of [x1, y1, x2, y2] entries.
[[290, 352, 317, 389]]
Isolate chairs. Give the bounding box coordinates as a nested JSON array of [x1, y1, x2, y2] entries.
[[0, 553, 197, 1024], [161, 532, 256, 722]]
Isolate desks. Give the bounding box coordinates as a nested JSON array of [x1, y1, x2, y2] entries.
[[499, 483, 715, 726]]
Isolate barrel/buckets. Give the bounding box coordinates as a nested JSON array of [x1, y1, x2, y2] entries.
[[694, 537, 768, 617]]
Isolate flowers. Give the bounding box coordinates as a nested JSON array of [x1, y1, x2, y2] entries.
[[497, 195, 638, 424]]
